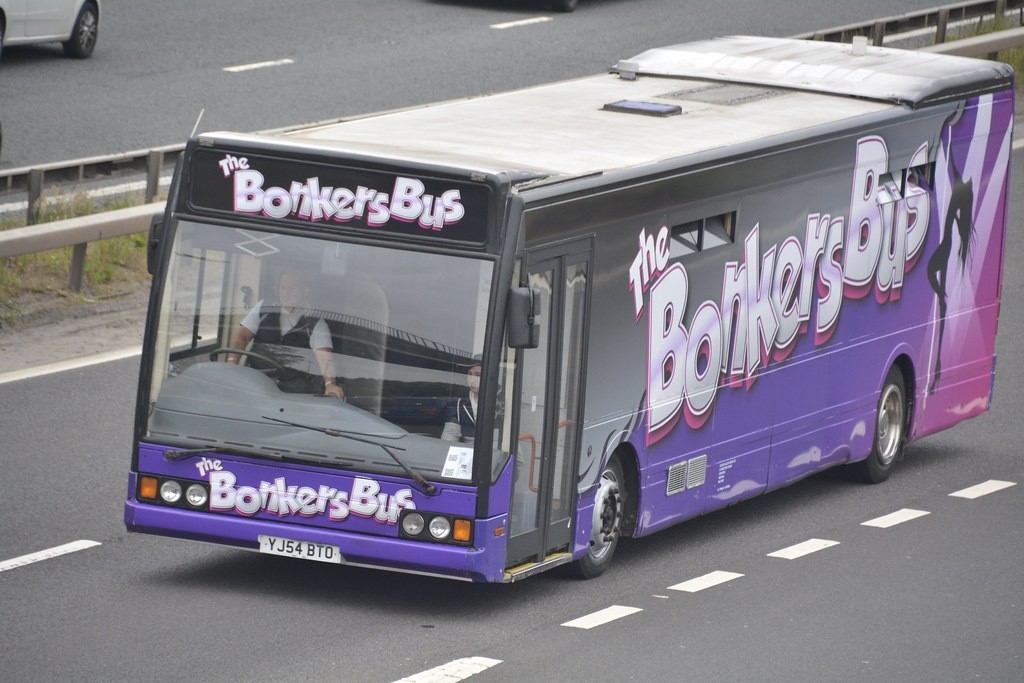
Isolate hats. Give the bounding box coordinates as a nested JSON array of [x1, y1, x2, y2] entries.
[[455, 354, 484, 367]]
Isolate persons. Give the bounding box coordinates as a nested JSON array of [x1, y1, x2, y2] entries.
[[433, 353, 505, 443], [226, 268, 344, 400]]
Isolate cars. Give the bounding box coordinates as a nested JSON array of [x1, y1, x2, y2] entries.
[[0, 0, 100, 57]]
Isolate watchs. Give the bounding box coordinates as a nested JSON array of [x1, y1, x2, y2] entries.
[[325, 380, 337, 386]]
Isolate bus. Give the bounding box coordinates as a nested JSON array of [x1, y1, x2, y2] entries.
[[123, 35, 1013, 584]]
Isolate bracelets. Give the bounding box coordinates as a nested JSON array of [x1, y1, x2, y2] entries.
[[227, 357, 238, 362]]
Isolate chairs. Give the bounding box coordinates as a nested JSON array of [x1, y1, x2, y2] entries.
[[340, 271, 389, 417]]
